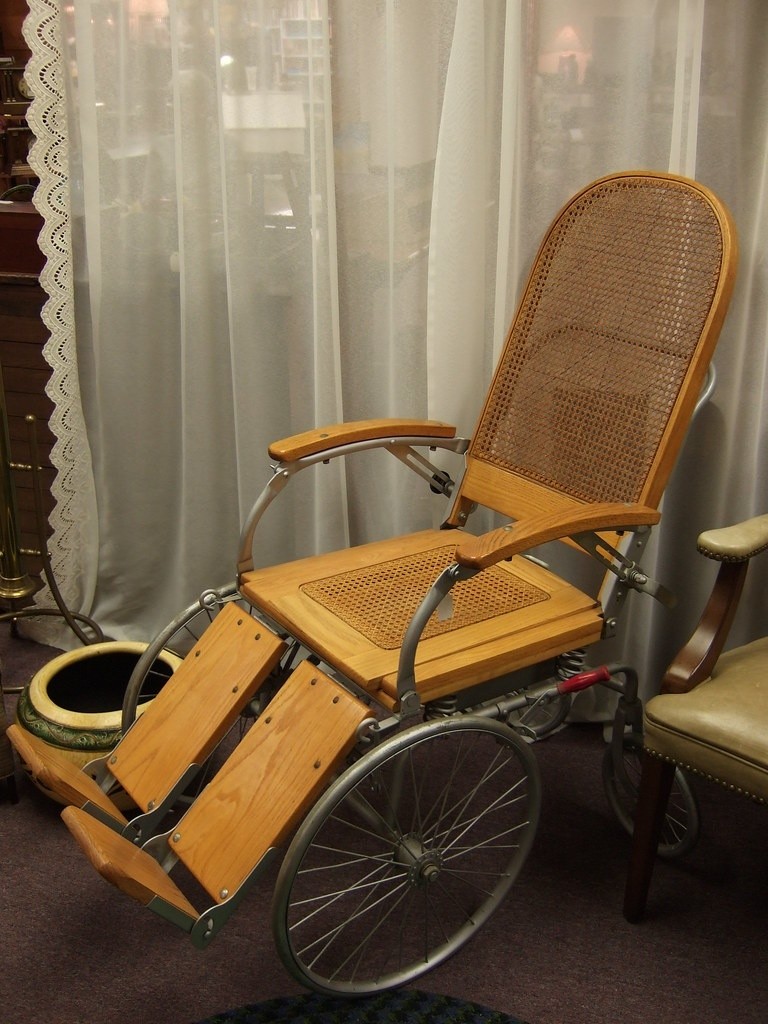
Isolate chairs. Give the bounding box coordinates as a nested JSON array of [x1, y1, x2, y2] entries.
[[622, 513, 768, 926]]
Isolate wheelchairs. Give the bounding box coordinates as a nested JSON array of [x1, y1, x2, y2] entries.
[[1, 167, 737, 998]]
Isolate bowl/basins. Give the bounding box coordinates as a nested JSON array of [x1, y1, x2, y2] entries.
[[15, 642, 184, 808]]
